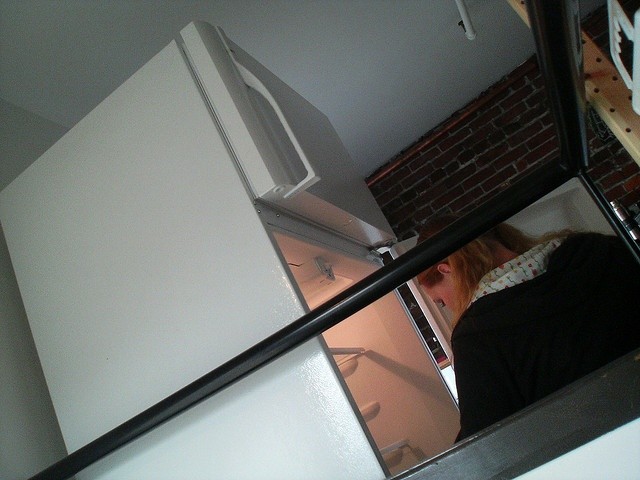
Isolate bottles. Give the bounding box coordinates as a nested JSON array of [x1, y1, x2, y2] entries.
[[607, 199, 640, 247]]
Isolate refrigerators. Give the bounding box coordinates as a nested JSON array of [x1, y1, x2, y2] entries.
[[1, 19, 489, 476]]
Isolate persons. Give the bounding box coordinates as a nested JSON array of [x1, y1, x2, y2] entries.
[[412, 210, 640, 445]]
